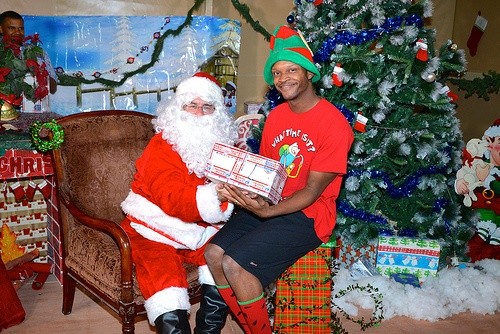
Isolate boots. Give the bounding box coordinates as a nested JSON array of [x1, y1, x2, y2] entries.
[[143, 286, 193, 334], [192, 265, 228, 334]]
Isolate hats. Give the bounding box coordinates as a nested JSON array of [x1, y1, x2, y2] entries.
[[263, 24, 322, 83], [176, 71, 226, 104]]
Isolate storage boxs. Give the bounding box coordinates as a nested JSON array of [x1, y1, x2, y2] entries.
[[375, 236, 440, 281], [205, 142, 288, 205]]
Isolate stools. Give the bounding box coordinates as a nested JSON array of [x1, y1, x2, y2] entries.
[[273, 232, 338, 334]]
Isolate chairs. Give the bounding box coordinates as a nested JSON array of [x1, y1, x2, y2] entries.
[[38, 110, 203, 334]]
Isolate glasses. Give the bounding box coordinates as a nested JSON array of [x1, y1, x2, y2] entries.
[[186, 103, 215, 115]]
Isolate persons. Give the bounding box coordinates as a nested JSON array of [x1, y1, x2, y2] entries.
[[0, 10, 58, 112], [118, 72, 267, 334], [204, 25, 355, 334]]
